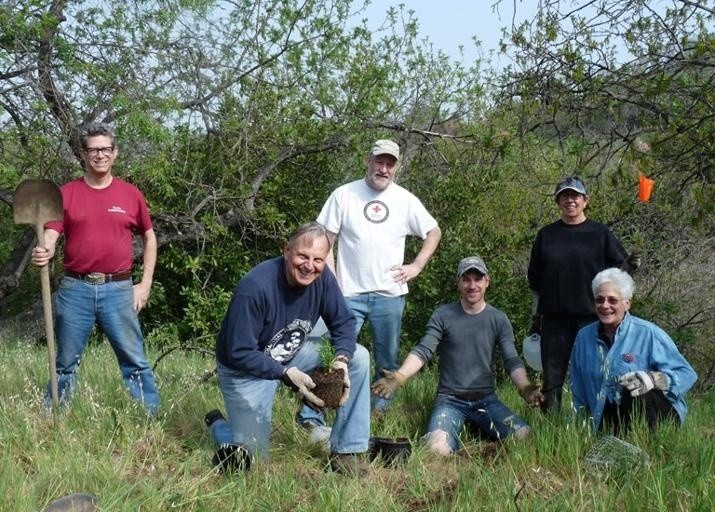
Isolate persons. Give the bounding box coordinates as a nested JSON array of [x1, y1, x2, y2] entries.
[[570, 266, 699, 446], [527, 177, 644, 423], [202, 221, 373, 482], [29, 122, 161, 428], [293, 139, 443, 434], [369, 255, 548, 461]]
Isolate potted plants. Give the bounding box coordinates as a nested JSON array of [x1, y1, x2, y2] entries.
[[305, 335, 345, 407], [370, 413, 410, 467]]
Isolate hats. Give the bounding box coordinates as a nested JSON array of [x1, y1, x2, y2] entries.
[[555, 179, 586, 202], [370, 139, 399, 161], [458, 256, 489, 278]]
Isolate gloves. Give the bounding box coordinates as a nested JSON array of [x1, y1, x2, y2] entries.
[[328, 355, 350, 408], [282, 366, 325, 413], [622, 249, 642, 274], [619, 371, 668, 397]]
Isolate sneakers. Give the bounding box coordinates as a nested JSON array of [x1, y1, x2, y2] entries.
[[204, 409, 225, 427], [324, 453, 370, 479]]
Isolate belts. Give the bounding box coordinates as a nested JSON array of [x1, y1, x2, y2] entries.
[[455, 393, 484, 402], [63, 269, 132, 285]]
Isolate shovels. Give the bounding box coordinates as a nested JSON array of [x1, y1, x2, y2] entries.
[[523, 293, 542, 372], [13, 178, 64, 431]]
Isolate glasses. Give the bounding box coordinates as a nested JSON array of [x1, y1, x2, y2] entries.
[[83, 144, 114, 156], [595, 297, 625, 306]]
